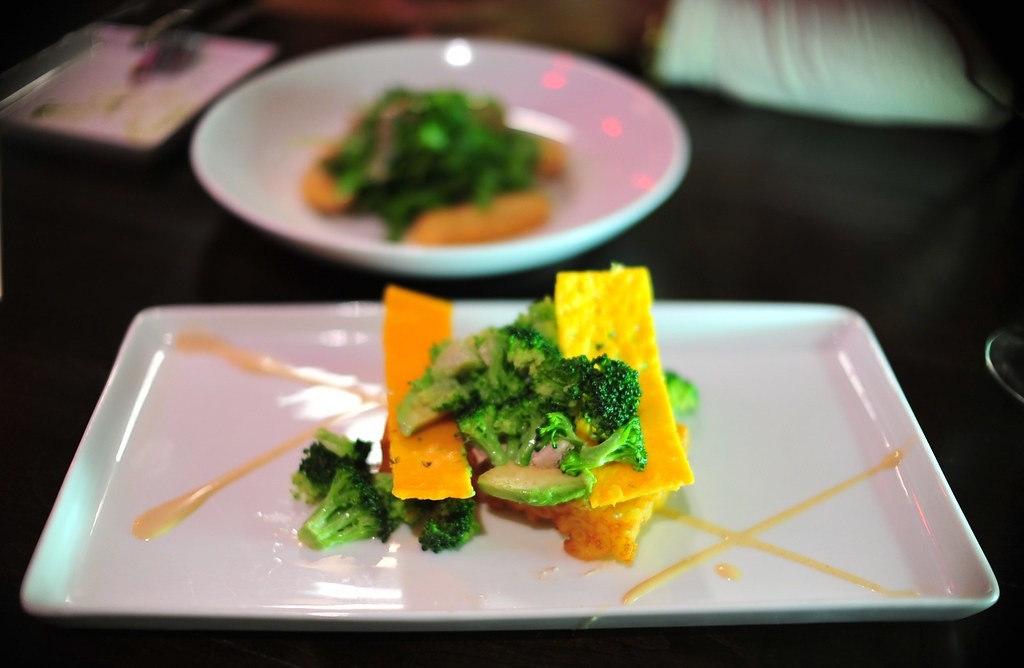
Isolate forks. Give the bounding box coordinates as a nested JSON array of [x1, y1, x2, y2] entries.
[[146, 5, 252, 66]]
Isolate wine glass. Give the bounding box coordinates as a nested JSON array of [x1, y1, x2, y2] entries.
[[981, 326, 1023, 399]]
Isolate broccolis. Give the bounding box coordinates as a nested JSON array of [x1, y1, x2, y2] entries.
[[293, 299, 701, 555], [322, 89, 539, 249]]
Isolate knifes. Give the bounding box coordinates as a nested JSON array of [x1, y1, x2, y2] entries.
[[132, 0, 202, 46], [1, 32, 104, 109]]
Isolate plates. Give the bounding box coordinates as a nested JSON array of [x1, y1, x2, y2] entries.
[[21, 300, 999, 630], [192, 37, 690, 276], [0, 24, 272, 149]]
